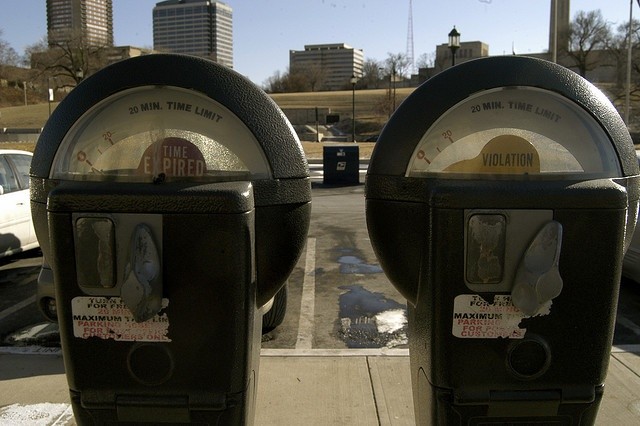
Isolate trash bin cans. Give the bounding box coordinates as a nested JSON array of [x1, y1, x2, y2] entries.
[[324, 146, 358, 188]]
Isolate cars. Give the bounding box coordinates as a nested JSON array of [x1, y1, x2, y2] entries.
[[0, 148, 41, 260]]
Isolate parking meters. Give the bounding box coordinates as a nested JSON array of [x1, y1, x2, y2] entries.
[[364, 53, 640, 426], [29, 52, 314, 426]]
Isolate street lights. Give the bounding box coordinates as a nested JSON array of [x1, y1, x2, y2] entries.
[[447, 23, 462, 66], [349, 74, 358, 143], [75, 65, 84, 85]]
